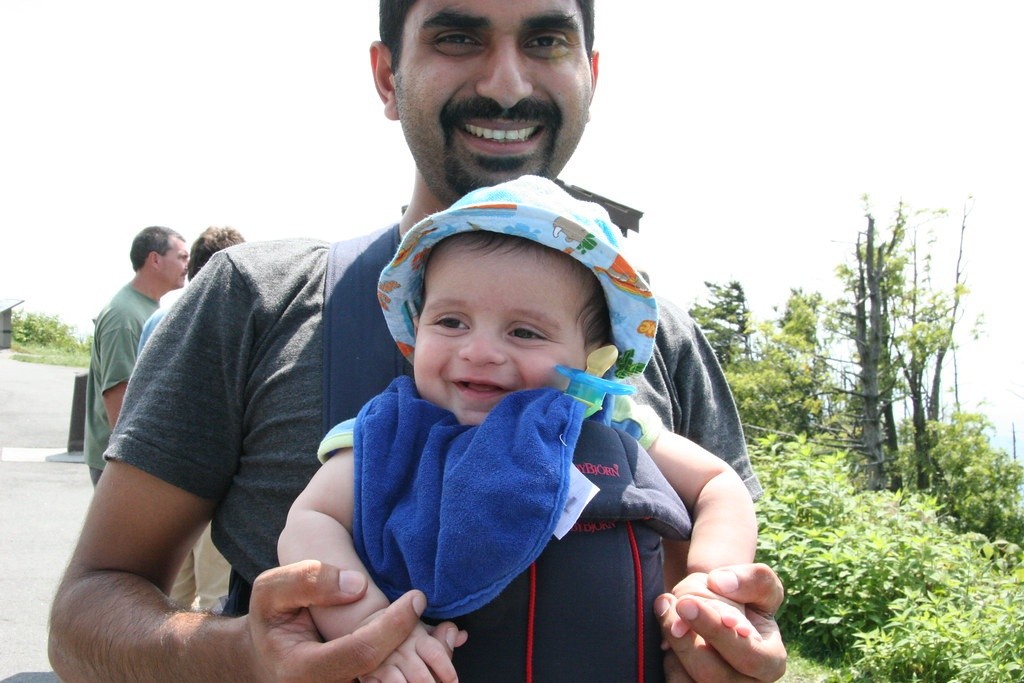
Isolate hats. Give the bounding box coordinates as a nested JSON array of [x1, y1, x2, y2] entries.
[[377, 176, 659, 384]]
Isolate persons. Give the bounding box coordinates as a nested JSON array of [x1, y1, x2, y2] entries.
[[46, 0, 787, 683], [275, 176, 757, 683], [80, 226, 247, 610]]
